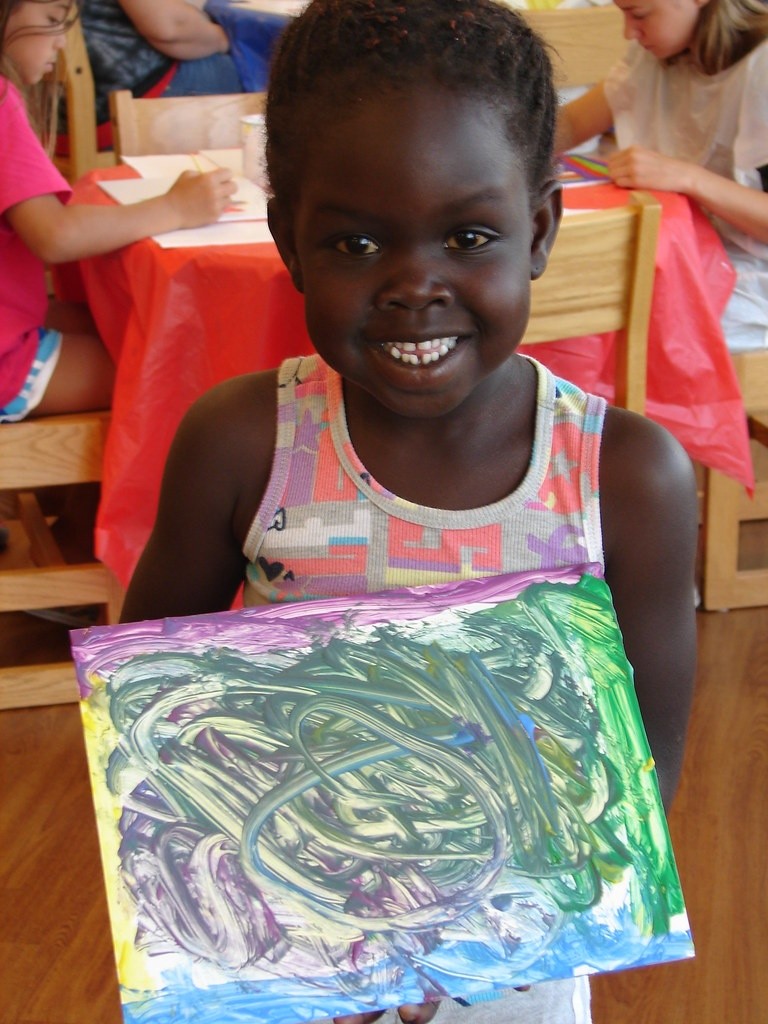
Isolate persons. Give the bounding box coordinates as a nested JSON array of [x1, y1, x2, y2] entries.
[[549, 0, 768, 607], [0, 0, 294, 627], [120, 0, 695, 1024]]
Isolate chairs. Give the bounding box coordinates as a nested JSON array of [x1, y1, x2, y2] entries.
[[1, 0, 767, 711]]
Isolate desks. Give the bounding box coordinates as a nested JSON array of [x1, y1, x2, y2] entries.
[[68, 154, 666, 622]]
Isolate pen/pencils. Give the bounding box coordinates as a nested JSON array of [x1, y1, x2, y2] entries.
[[190, 154, 205, 174]]
